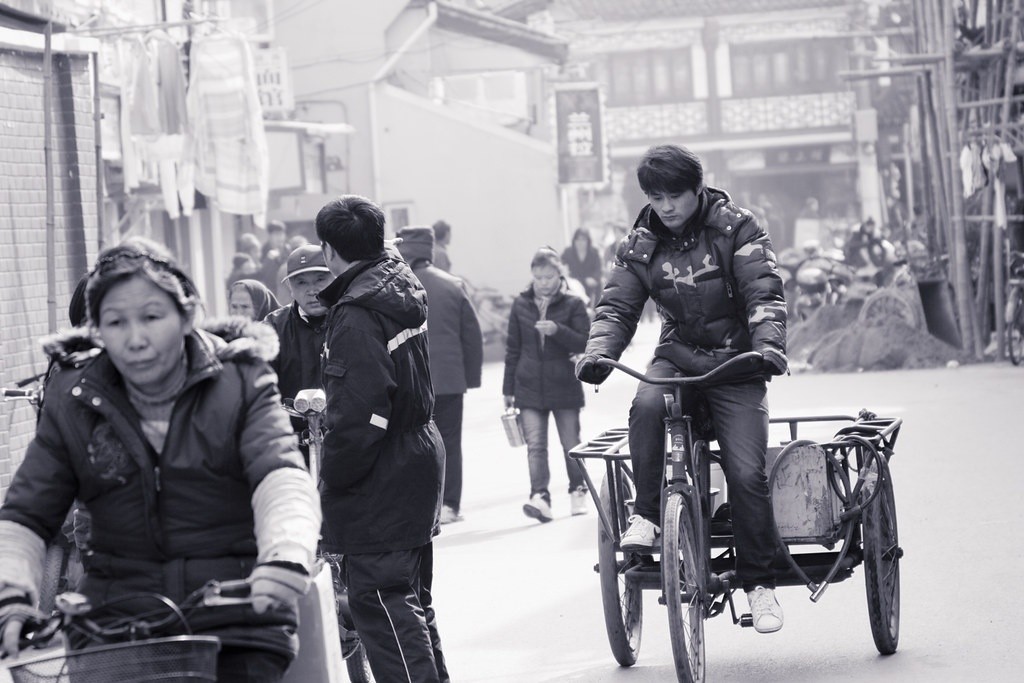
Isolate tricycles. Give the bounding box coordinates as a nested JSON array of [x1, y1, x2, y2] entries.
[[568, 351, 904, 683]]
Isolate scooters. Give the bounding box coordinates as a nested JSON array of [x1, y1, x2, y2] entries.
[[2, 369, 376, 683], [792, 241, 888, 321]]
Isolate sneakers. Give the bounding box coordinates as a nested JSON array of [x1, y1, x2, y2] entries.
[[748, 584, 782, 633], [524, 497, 553, 523], [620, 513, 662, 549], [571, 486, 586, 516]]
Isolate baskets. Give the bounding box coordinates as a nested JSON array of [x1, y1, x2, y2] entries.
[[7, 634, 221, 683]]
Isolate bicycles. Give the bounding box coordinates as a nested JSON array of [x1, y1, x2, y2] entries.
[[0, 575, 254, 683], [1006, 251, 1024, 365]]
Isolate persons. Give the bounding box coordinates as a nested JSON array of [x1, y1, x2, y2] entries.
[[500, 245, 592, 524], [849, 218, 877, 259], [0, 239, 322, 683], [575, 144, 787, 633], [227, 278, 281, 323], [313, 195, 447, 683], [799, 198, 819, 219], [260, 244, 338, 471], [431, 219, 452, 272], [390, 226, 483, 525], [223, 219, 308, 306], [561, 226, 603, 300]]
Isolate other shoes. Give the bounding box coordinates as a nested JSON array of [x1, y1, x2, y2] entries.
[[439, 506, 457, 523]]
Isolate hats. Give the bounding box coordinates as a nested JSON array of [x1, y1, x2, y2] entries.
[[396, 228, 434, 269], [281, 245, 330, 284]]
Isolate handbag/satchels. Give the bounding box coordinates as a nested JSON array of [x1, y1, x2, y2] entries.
[[500, 406, 525, 447]]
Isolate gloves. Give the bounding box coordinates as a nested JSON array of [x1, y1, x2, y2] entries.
[[576, 355, 610, 384], [749, 345, 787, 377], [248, 566, 311, 613], [0, 603, 50, 659]]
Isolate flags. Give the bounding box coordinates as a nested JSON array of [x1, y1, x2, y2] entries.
[[552, 84, 609, 192]]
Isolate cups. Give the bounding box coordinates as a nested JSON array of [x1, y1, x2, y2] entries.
[[501, 405, 526, 448]]
